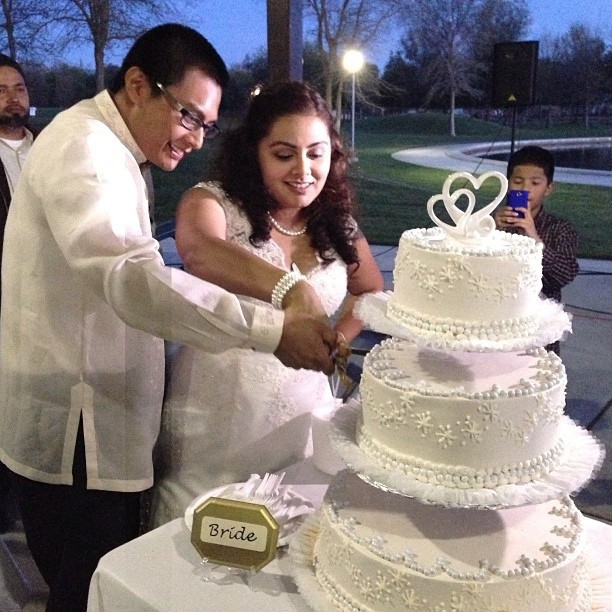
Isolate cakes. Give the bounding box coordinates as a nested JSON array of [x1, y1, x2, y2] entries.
[[311, 467, 595, 611], [355, 335, 567, 489], [386, 172, 545, 343]]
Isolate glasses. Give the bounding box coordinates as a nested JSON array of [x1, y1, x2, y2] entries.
[[157, 82, 222, 140]]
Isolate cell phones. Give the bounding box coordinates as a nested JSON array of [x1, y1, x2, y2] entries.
[[506, 190, 527, 218]]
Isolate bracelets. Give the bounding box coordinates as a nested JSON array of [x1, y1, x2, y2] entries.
[[269, 272, 310, 310]]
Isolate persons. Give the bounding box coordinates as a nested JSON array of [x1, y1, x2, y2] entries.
[[139, 81, 383, 533], [1, 55, 37, 315], [489, 146, 582, 357], [0, 23, 339, 610]]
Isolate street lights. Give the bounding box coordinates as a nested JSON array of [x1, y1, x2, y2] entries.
[[342, 50, 364, 150]]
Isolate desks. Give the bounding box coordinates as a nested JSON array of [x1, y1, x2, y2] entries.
[[87, 454, 612, 610]]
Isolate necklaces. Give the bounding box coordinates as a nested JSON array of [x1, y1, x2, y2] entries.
[[266, 206, 311, 236]]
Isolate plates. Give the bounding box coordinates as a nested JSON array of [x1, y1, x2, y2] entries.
[[185, 483, 315, 549]]
